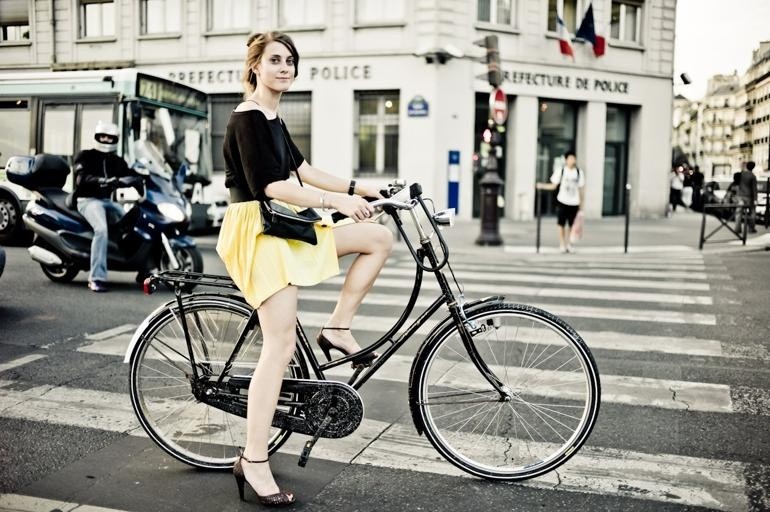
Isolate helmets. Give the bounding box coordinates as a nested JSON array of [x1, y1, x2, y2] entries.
[[90, 120, 121, 155]]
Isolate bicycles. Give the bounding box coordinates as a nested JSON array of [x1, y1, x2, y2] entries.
[[123, 180, 600, 486]]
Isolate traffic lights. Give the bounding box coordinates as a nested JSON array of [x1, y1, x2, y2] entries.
[[477, 35, 501, 89]]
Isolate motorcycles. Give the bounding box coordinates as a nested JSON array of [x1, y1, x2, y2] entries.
[[10, 137, 209, 294]]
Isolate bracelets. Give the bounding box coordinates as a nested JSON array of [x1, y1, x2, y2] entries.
[[348, 179, 355, 196], [320, 189, 330, 214]]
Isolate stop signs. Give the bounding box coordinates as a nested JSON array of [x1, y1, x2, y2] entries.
[[490, 89, 509, 124]]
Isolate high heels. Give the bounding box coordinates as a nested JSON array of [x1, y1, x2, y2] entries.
[[233, 450, 297, 509], [316, 326, 378, 366]]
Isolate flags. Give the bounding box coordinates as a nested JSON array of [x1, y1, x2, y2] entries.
[[576, 4, 595, 45], [593, 11, 606, 57], [556, 14, 576, 63]]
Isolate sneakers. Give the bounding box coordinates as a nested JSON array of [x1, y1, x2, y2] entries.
[[87, 279, 109, 293]]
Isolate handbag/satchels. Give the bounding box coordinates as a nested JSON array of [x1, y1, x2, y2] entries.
[[570, 209, 584, 244], [256, 197, 323, 245]]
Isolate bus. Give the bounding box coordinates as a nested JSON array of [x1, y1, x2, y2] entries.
[[0, 71, 211, 242]]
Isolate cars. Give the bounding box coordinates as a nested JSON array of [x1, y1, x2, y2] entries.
[[704, 169, 768, 220], [204, 188, 232, 231]]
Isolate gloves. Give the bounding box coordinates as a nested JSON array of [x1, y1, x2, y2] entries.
[[99, 176, 120, 188]]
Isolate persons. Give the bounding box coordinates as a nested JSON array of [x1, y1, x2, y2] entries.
[[72, 118, 141, 293], [215, 32, 393, 508], [536, 151, 586, 253], [671, 161, 760, 232]]
